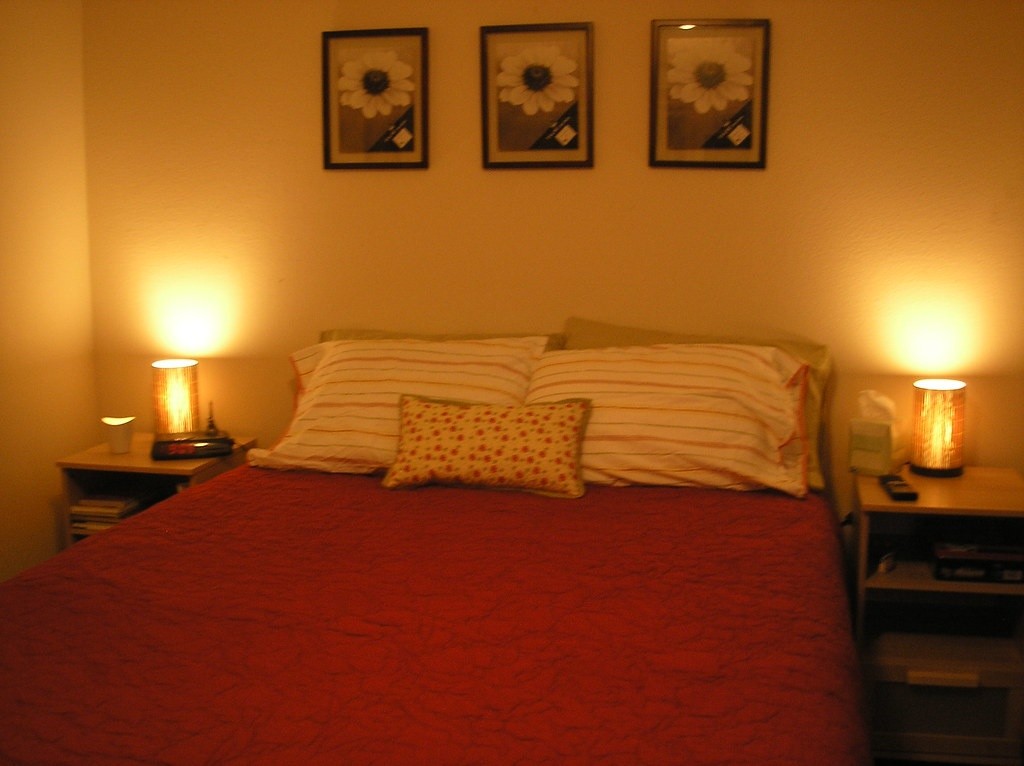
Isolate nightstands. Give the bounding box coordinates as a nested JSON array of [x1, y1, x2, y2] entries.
[[854, 467, 1024, 766], [54, 431, 257, 548]]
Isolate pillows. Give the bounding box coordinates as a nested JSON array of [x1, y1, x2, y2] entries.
[[249, 318, 832, 498]]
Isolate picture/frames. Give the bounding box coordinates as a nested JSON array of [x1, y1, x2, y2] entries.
[[322, 27, 429, 169], [480, 21, 595, 169], [649, 19, 771, 169]]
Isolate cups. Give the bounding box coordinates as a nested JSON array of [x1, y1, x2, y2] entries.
[[100, 416, 136, 454]]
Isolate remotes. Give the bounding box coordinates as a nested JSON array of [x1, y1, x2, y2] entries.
[[879, 475, 922, 503]]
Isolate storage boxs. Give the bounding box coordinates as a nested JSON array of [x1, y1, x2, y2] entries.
[[929, 539, 1024, 582]]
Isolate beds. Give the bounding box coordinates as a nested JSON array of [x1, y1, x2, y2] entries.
[[0, 461, 876, 766]]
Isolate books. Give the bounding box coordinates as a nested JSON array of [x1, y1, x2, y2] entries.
[[70, 491, 153, 535]]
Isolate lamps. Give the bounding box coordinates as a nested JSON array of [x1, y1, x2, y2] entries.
[[152, 359, 199, 434], [908, 378, 966, 477]]
